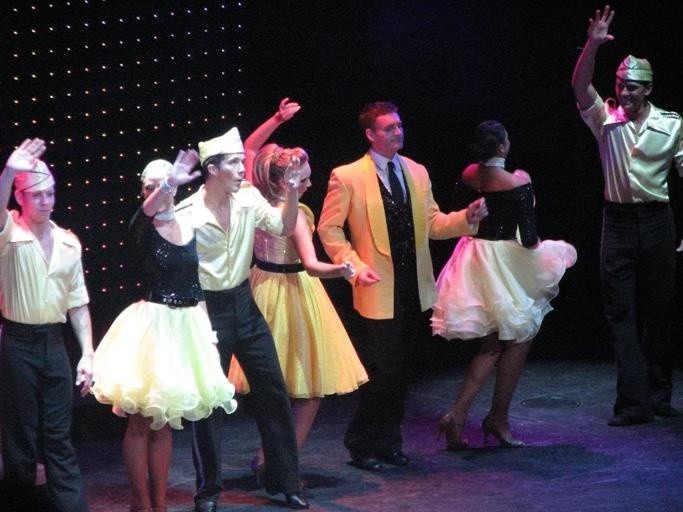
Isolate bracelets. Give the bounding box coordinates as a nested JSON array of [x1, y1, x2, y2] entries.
[[159, 176, 177, 194]]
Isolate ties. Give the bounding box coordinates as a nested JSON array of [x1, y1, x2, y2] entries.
[[385, 162, 405, 206]]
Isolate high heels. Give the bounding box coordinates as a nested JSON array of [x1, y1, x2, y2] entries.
[[482, 416, 526, 449], [251, 455, 267, 489], [436, 413, 470, 452]]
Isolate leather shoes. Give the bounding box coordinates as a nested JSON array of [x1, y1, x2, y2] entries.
[[268, 486, 309, 510], [607, 412, 632, 427], [194, 500, 217, 512], [654, 402, 680, 417], [344, 443, 381, 471], [374, 446, 409, 466]]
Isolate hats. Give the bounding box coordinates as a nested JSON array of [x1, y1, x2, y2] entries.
[[198, 127, 245, 166], [615, 54, 652, 82], [13, 160, 56, 193]]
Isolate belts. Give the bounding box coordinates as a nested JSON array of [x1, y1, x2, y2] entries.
[[143, 290, 199, 309]]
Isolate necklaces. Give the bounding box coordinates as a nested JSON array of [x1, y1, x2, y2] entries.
[[484, 156, 506, 168], [153, 206, 177, 221]]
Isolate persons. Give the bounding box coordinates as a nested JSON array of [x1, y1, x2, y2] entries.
[[318, 102, 488, 470], [179, 127, 311, 509], [431, 119, 577, 452], [227, 96, 350, 481], [572, 3, 683, 426], [87, 147, 237, 512], [0, 137, 94, 510]]
